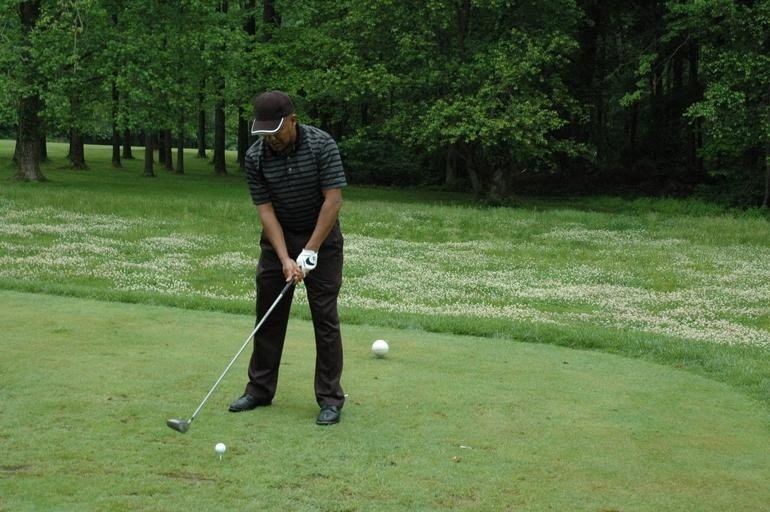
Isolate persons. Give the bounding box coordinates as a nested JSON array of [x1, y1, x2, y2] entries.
[[225, 89, 347, 426]]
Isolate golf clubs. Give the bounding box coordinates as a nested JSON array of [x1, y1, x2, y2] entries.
[[167, 270, 301, 432]]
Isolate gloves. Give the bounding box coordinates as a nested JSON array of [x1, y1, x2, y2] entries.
[[295, 247, 319, 282]]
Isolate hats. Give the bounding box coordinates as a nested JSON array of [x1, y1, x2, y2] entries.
[[251, 87, 293, 135]]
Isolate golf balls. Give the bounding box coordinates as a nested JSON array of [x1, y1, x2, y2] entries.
[[215, 443, 226, 454], [372, 340, 388, 356]]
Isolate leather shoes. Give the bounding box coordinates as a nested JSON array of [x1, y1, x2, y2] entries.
[[228, 389, 272, 414], [316, 398, 345, 424]]
